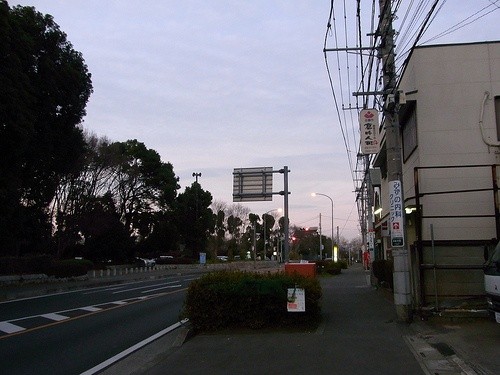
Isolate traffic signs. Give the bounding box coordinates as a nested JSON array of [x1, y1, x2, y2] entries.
[[388, 180, 405, 248]]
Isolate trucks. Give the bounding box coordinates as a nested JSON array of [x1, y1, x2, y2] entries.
[[482, 236, 500, 324]]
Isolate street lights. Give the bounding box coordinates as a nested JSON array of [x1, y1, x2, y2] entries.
[[264, 208, 283, 261], [191, 172, 201, 217], [312, 192, 334, 262]]
